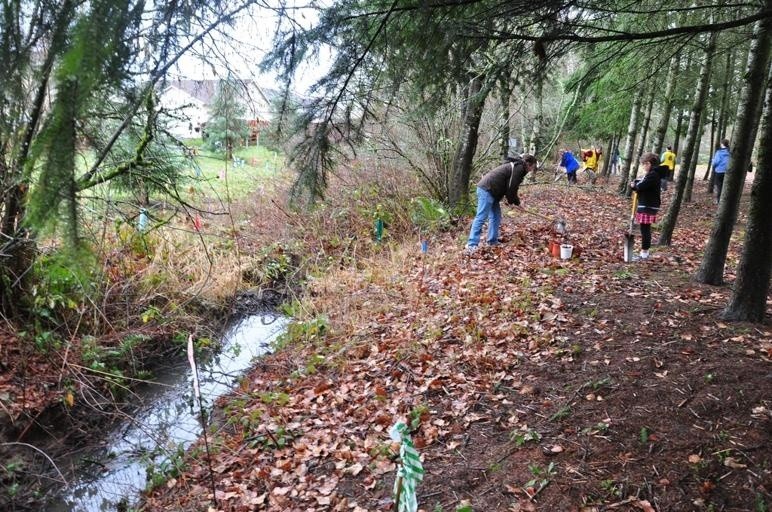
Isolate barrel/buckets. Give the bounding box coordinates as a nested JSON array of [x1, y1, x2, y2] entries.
[[560, 244, 573, 258]]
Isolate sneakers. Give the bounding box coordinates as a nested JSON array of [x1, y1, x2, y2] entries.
[[485, 241, 502, 247], [640, 250, 649, 259], [465, 244, 479, 253]]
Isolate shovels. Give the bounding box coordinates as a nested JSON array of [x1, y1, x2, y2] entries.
[[623, 191, 638, 263]]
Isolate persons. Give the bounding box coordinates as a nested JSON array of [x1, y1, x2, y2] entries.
[[558, 145, 620, 185], [630, 152, 669, 258], [712, 139, 732, 201], [465, 153, 536, 250], [661, 146, 678, 191]]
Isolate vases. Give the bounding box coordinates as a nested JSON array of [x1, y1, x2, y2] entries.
[[548, 237, 573, 259]]
